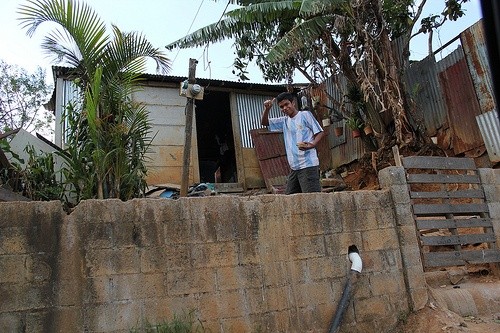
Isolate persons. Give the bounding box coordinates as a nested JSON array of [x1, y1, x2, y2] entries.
[[261, 92, 325, 194]]
